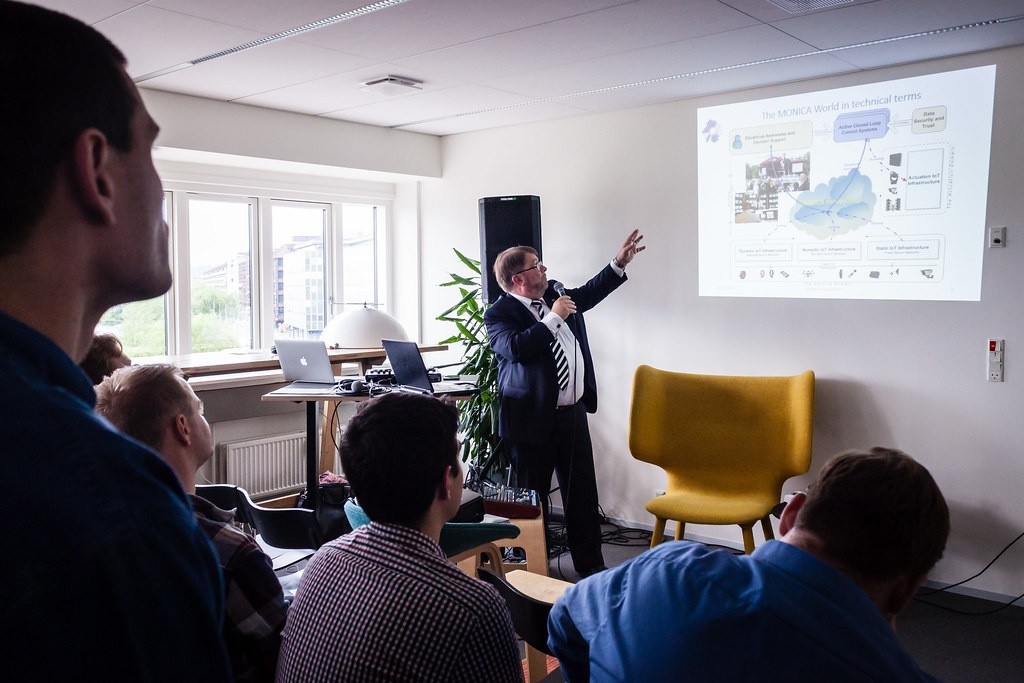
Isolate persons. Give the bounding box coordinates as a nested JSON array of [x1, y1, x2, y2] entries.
[[734, 174, 810, 223], [0, 0, 228, 683], [80, 335, 131, 383], [548, 445, 950, 683], [277, 391, 527, 682], [86, 366, 288, 683], [485, 229, 646, 580]]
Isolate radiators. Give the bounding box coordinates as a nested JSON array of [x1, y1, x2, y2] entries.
[[216, 430, 305, 501]]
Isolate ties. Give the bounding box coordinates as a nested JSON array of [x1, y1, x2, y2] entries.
[[530, 299, 569, 391]]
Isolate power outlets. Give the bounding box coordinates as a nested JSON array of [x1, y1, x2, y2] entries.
[[987, 337, 1005, 383]]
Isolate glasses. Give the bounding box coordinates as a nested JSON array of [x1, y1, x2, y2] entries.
[[513, 261, 543, 275]]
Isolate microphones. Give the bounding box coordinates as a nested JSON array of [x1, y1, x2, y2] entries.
[[554, 282, 575, 316], [350, 382, 385, 392]]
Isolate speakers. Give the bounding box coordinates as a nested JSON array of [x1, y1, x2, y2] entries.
[[478, 195, 542, 305]]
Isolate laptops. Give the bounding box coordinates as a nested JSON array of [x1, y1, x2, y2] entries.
[[273, 338, 367, 387], [381, 339, 480, 397]]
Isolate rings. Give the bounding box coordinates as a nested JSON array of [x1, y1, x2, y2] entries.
[[635, 243, 637, 246]]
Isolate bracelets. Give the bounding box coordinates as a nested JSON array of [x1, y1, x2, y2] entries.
[[613, 256, 625, 269]]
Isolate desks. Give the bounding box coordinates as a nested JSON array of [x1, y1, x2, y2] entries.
[[259, 376, 477, 513]]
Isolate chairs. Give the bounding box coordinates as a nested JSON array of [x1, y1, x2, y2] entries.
[[198, 478, 575, 683], [628, 364, 814, 554]]
